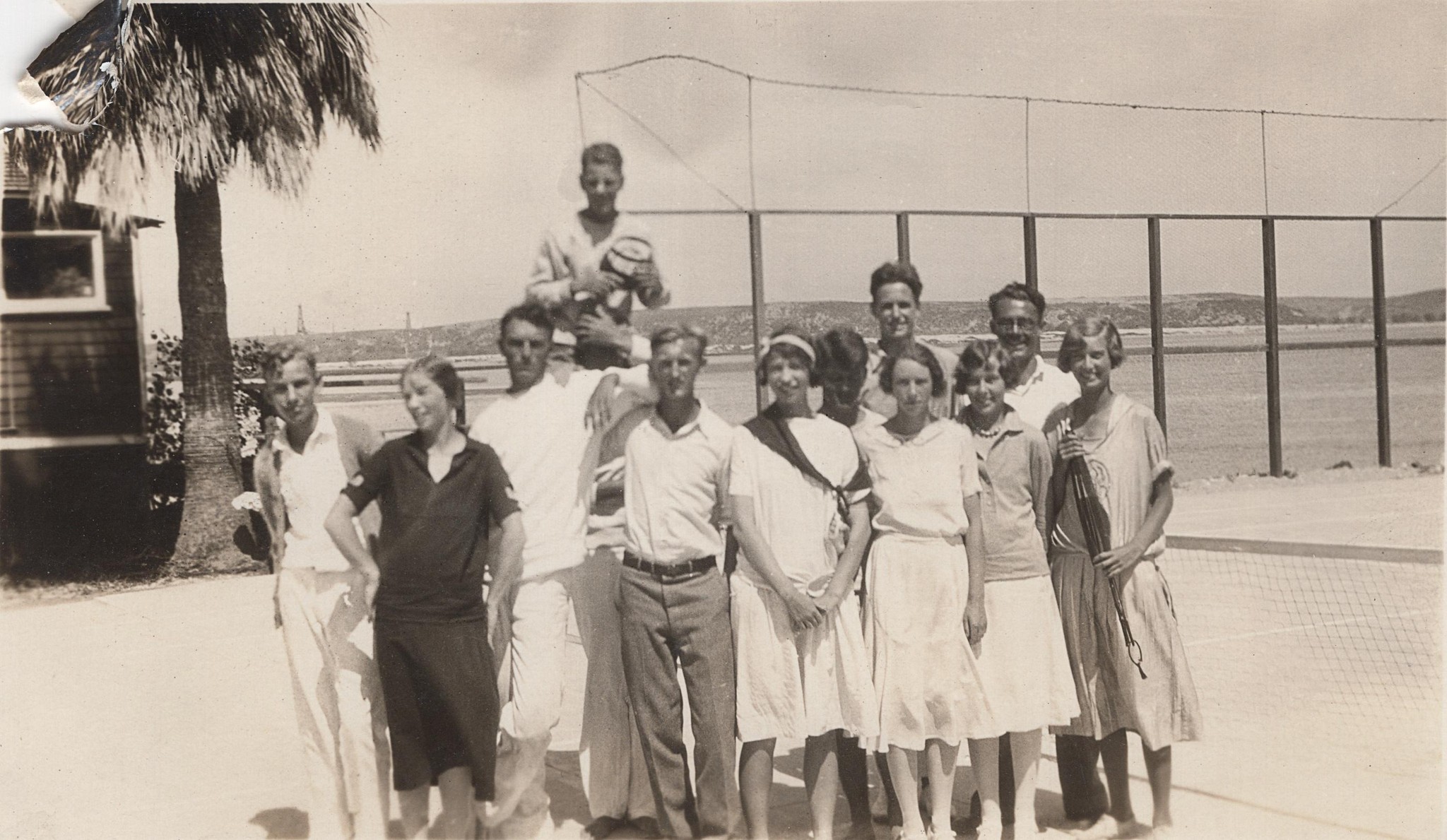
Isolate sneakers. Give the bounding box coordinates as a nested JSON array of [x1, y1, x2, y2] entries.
[[1078, 814, 1138, 839], [1152, 822, 1175, 839]]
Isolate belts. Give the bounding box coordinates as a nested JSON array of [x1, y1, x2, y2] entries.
[[624, 551, 717, 576]]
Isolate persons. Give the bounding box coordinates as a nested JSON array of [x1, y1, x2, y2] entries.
[[569, 305, 655, 369], [472, 303, 650, 840], [812, 325, 887, 460], [980, 281, 1080, 428], [954, 340, 1080, 840], [598, 325, 739, 839], [527, 143, 667, 324], [867, 262, 962, 419], [1041, 317, 1207, 840], [325, 355, 526, 840], [732, 330, 872, 840], [856, 348, 987, 840], [252, 342, 387, 840]]
